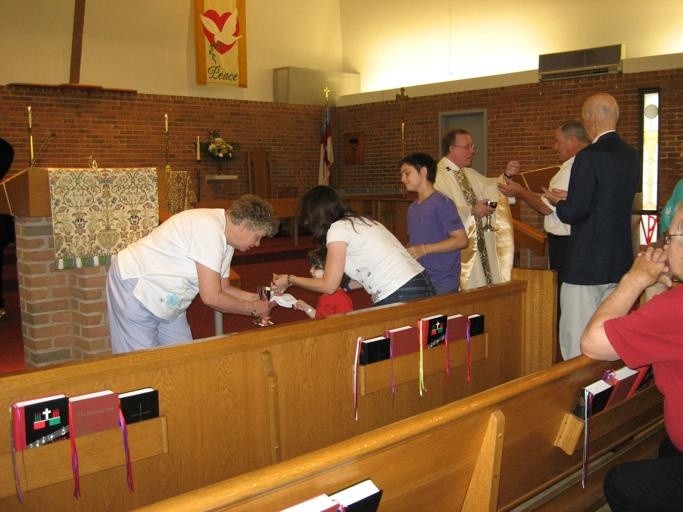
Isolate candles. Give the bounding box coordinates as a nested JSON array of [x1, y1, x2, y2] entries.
[[163, 111, 171, 137], [400, 122, 406, 141], [195, 134, 201, 161], [27, 106, 36, 164]]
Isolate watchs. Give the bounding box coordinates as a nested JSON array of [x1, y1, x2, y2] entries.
[[250, 302, 257, 318], [286, 273, 291, 284]]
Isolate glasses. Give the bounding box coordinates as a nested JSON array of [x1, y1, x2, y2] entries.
[[451, 143, 479, 151], [663, 230, 683, 241]]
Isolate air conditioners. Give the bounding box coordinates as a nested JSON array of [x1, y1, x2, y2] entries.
[[537, 42, 626, 82]]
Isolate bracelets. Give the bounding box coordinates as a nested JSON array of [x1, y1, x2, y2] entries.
[[305, 307, 315, 315], [422, 242, 427, 259], [503, 171, 512, 180], [343, 281, 353, 292]]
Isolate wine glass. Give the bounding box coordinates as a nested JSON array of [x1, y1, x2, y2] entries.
[[252, 285, 276, 327], [482, 202, 498, 232]]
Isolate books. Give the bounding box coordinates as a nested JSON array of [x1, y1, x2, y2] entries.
[[628, 359, 653, 402], [359, 334, 390, 366], [573, 377, 613, 420], [387, 324, 418, 360], [418, 314, 447, 352], [68, 389, 119, 438], [468, 312, 486, 337], [605, 365, 640, 413], [328, 474, 384, 512], [447, 314, 468, 343], [11, 393, 69, 451], [280, 491, 340, 512], [118, 385, 159, 427]]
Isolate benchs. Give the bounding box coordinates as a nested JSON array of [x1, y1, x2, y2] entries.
[[127, 344, 667, 511], [0, 264, 560, 511]]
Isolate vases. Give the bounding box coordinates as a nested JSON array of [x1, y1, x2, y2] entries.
[[214, 153, 227, 177]]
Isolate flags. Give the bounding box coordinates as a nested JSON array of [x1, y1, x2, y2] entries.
[[317, 95, 337, 186]]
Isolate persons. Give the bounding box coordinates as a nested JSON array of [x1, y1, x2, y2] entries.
[[269, 185, 435, 306], [434, 128, 520, 291], [579, 199, 683, 512], [104, 193, 279, 355], [294, 248, 354, 321], [496, 119, 591, 327], [399, 152, 470, 295], [541, 92, 639, 363]]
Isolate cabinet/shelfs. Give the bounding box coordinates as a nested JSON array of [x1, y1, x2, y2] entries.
[[208, 174, 238, 181]]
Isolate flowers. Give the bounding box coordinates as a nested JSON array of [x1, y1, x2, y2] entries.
[[190, 126, 243, 161]]
